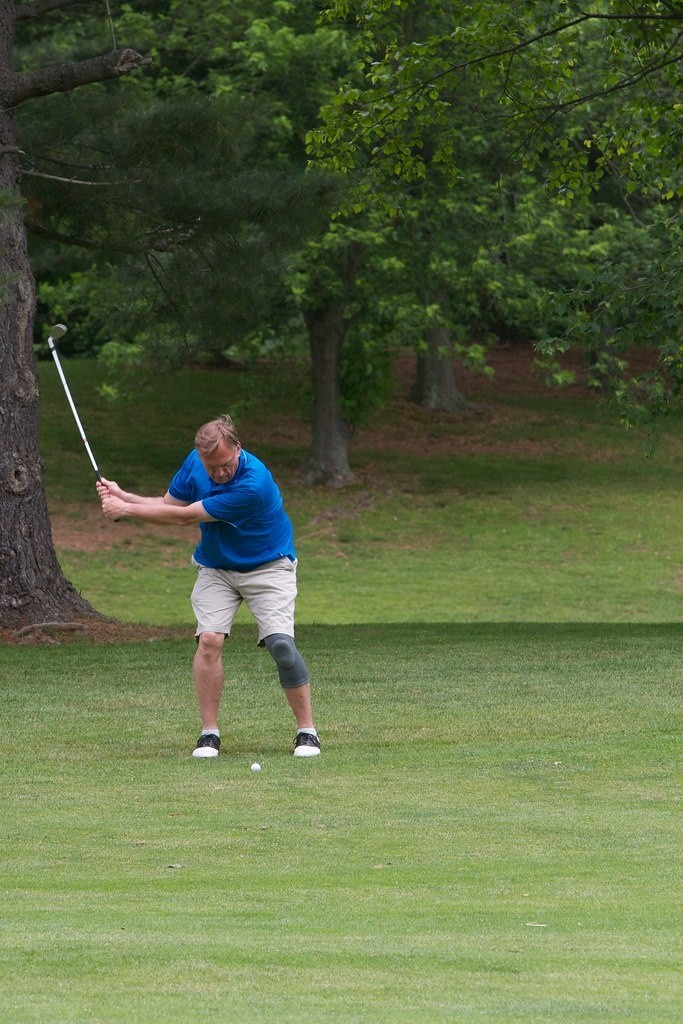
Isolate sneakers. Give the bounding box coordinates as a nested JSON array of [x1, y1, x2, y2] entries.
[[292, 732, 321, 757], [193, 734, 222, 758]]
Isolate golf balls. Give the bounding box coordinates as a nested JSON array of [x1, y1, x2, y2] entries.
[[251, 763, 261, 771]]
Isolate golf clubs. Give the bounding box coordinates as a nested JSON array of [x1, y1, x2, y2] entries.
[[47, 324, 121, 523]]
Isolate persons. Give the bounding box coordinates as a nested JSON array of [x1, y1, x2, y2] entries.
[[95, 416, 321, 757]]
